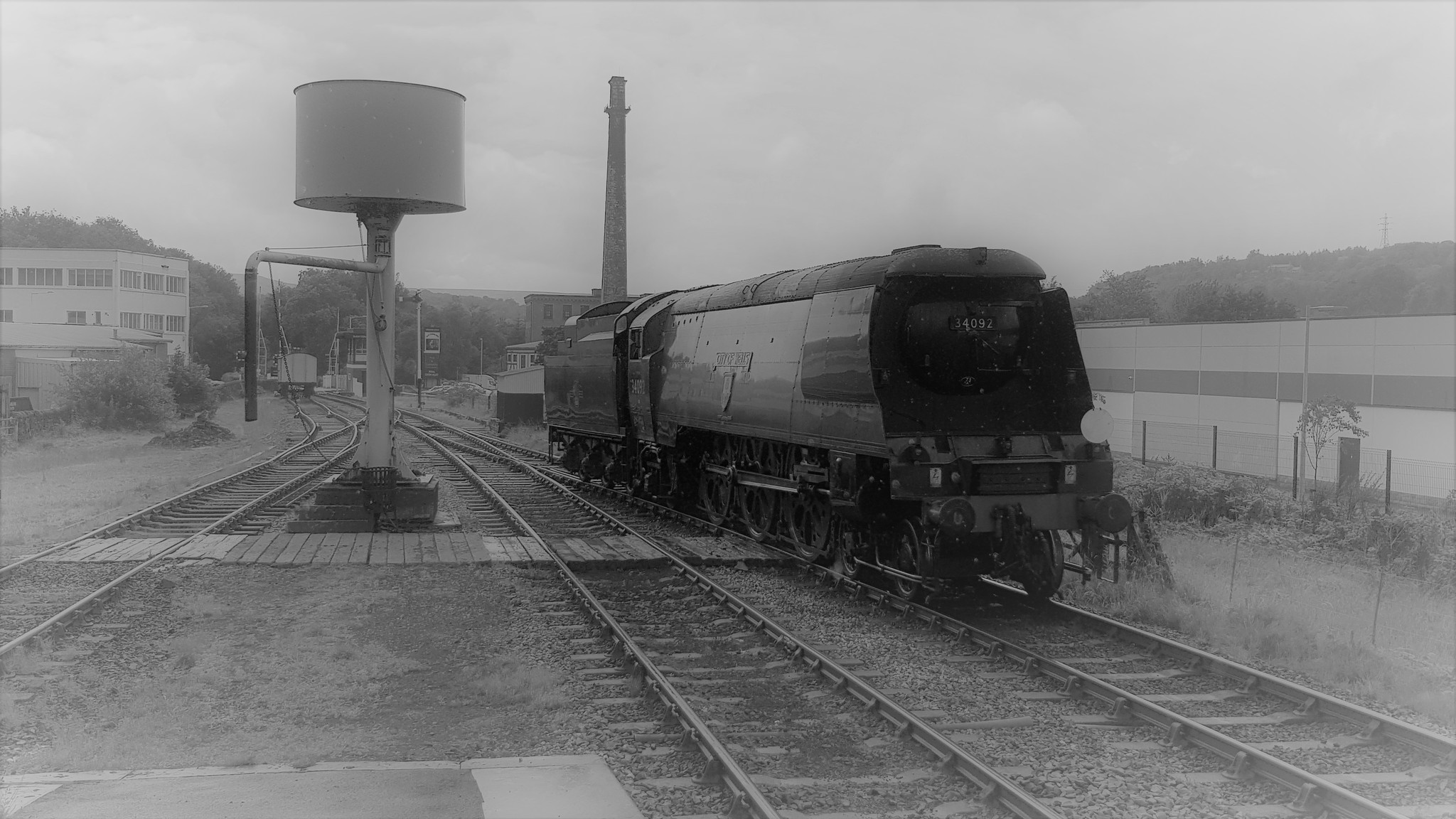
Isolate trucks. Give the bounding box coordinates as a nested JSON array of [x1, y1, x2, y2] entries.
[[462, 374, 497, 391]]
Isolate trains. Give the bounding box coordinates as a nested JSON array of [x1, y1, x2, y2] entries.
[[545, 243, 1134, 603], [275, 350, 318, 398]]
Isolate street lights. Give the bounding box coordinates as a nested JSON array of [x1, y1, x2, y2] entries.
[[1298, 303, 1350, 501]]
